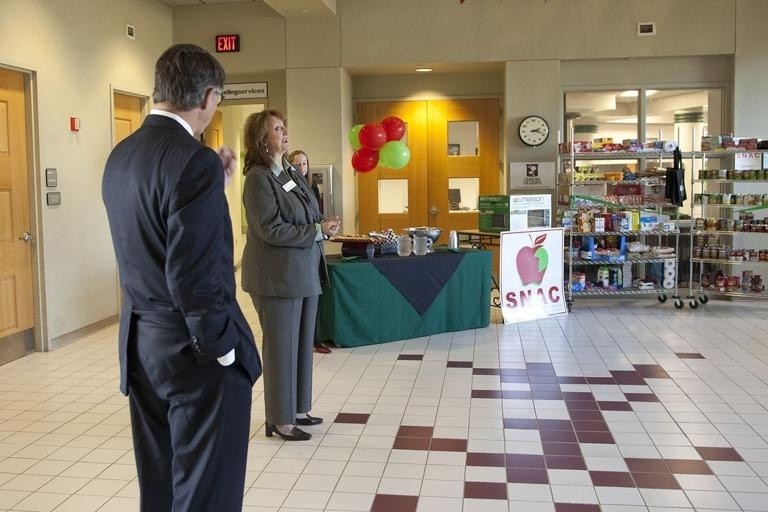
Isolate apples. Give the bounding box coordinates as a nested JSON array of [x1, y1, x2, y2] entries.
[[515, 246, 548, 285]]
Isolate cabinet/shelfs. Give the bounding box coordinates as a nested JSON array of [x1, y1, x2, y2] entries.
[[702, 154, 767, 296], [558, 149, 725, 214], [565, 228, 678, 298]]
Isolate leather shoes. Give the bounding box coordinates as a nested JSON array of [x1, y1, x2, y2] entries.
[[316, 342, 333, 353]]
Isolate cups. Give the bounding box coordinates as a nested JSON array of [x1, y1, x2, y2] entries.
[[397, 237, 415, 257], [414, 236, 433, 255]]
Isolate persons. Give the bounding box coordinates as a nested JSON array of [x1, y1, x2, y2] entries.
[[103, 43, 266, 512], [286, 148, 339, 353], [238, 109, 341, 442]]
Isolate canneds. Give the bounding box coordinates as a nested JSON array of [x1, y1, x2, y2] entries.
[[695, 212, 768, 232], [694, 237, 768, 262], [701, 270, 763, 292], [694, 193, 768, 205], [699, 169, 768, 180]]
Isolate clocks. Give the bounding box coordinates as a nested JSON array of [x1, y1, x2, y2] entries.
[[518, 115, 549, 146]]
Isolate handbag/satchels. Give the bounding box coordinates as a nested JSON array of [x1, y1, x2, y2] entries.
[[664, 147, 687, 206]]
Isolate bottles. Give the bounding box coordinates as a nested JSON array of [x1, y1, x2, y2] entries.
[[448, 231, 457, 249]]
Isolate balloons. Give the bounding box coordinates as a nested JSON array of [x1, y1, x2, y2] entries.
[[350, 116, 410, 175]]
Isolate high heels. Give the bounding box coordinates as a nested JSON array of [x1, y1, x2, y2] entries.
[[296, 412, 322, 426], [265, 421, 311, 440]]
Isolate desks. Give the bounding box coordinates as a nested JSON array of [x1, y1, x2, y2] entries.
[[457, 229, 499, 310], [317, 247, 493, 347]]
